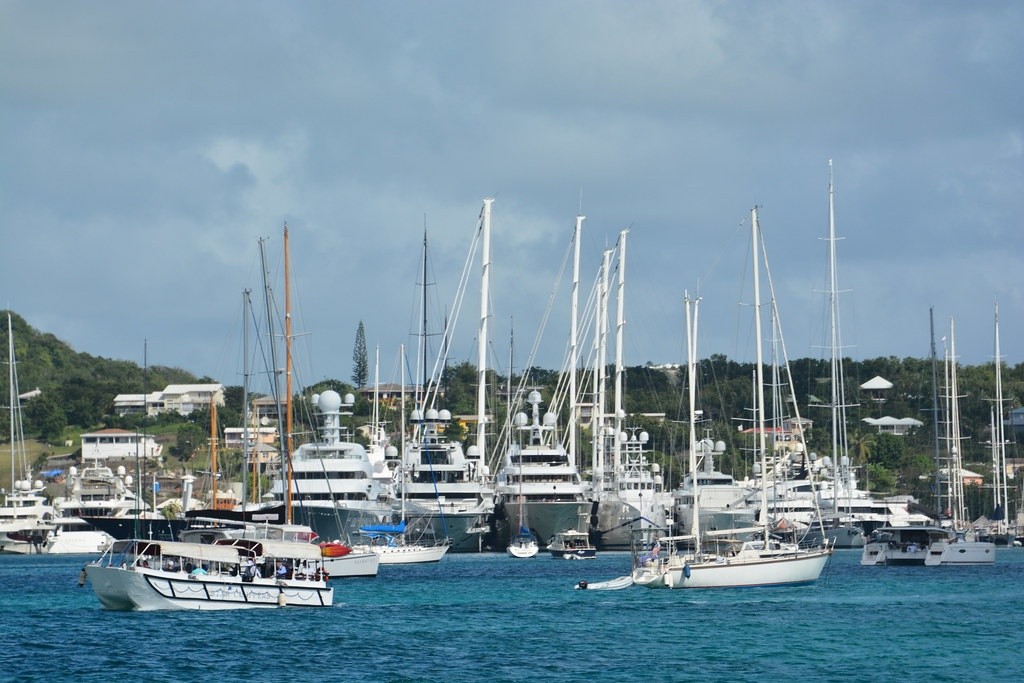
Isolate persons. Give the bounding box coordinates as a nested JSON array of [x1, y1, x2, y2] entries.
[[109, 550, 319, 582], [647, 553, 671, 574]]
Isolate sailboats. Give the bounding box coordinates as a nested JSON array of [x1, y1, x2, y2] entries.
[[592, 192, 1023, 566], [573, 207, 833, 591], [1, 188, 592, 578]]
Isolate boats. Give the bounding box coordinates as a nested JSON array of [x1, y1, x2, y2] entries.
[[85, 538, 336, 614], [546, 527, 598, 560]]
